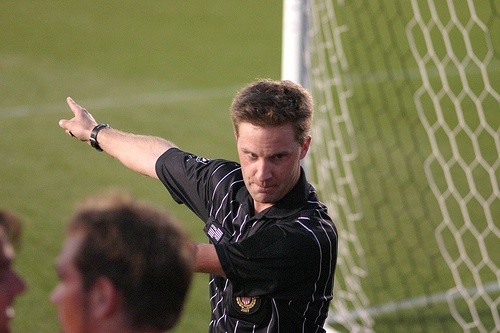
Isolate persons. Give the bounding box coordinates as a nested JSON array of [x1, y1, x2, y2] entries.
[[58, 79, 340, 333], [46, 187, 195, 333], [0, 207, 27, 332]]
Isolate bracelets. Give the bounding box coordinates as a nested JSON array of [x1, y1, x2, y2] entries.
[[88, 122, 110, 152]]
